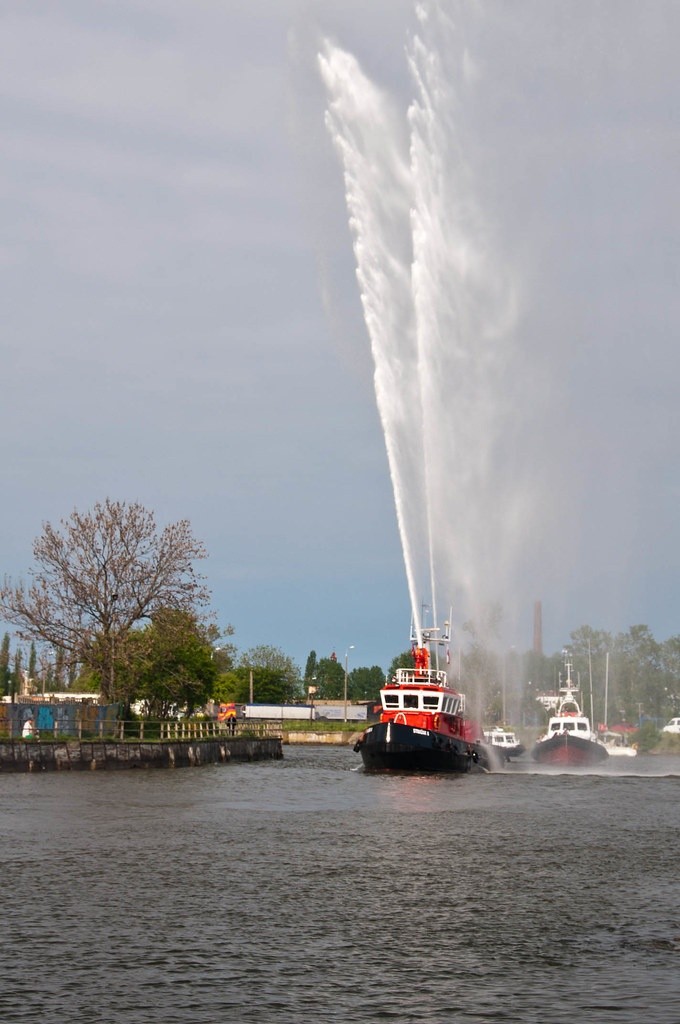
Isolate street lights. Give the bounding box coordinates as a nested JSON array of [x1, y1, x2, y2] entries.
[[344, 646, 355, 723], [211, 647, 220, 661]]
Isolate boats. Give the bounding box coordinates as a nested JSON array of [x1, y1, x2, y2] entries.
[[352, 595, 490, 773], [484, 724, 526, 759], [530, 650, 609, 765]]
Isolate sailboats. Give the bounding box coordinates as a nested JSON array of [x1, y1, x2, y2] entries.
[[585, 639, 637, 756]]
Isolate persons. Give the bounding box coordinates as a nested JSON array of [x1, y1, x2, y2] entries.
[[22, 717, 39, 740], [416, 645, 428, 677], [227, 714, 237, 736], [632, 742, 639, 749], [552, 729, 569, 739]]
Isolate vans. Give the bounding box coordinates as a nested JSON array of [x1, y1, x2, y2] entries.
[[663, 717, 680, 733]]
